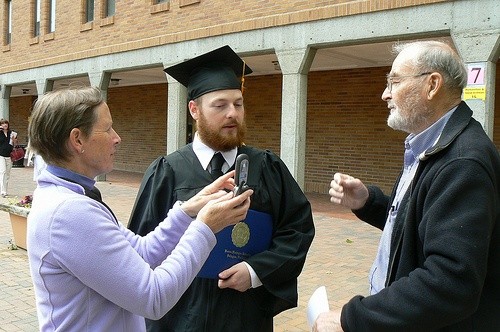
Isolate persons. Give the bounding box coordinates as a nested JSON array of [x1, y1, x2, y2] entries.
[[26, 88, 253, 332], [0, 119, 16, 198], [27, 150, 47, 182], [312, 41, 500, 332], [127, 45, 315, 332]]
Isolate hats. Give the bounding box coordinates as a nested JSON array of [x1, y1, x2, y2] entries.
[[163, 45, 253, 101]]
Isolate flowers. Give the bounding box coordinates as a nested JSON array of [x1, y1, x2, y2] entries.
[[7, 195, 33, 208]]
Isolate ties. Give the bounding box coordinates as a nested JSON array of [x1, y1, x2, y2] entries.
[[209, 153, 225, 181]]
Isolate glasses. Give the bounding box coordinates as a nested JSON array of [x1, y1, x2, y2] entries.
[[386, 72, 434, 92]]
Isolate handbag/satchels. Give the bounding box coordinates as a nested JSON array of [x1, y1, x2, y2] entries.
[[10, 140, 24, 162]]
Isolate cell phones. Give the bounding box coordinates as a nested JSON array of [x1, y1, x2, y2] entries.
[[232, 154, 249, 208]]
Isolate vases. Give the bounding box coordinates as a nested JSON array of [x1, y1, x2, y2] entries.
[[0, 202, 30, 250]]
